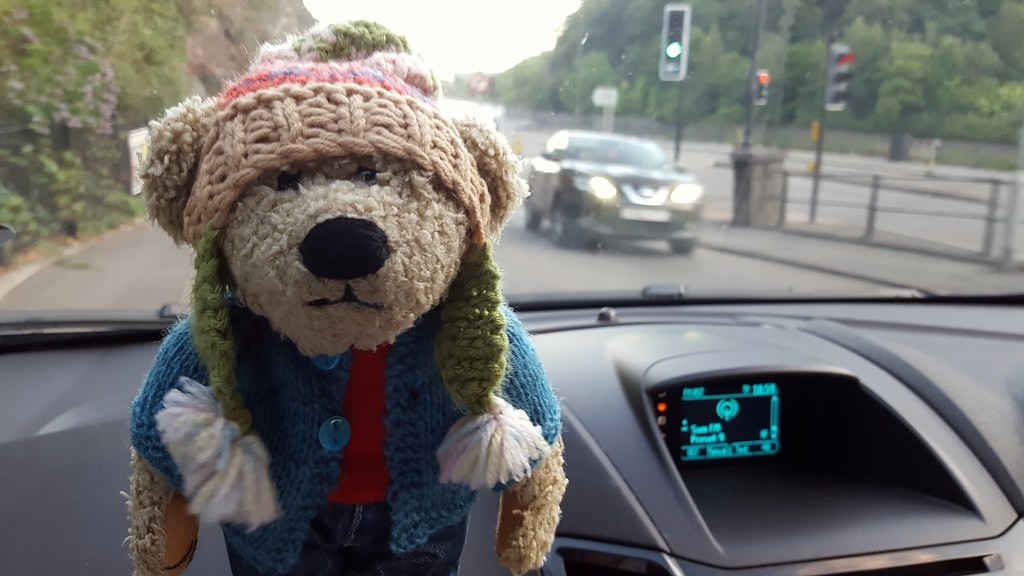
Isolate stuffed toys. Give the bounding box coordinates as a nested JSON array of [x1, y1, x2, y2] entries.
[[122, 17, 568, 576]]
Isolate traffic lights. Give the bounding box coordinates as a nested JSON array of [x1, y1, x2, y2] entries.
[[753, 69, 770, 106], [660, 5, 690, 81], [824, 44, 856, 112]]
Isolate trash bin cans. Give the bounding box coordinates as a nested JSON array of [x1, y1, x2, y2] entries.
[[889, 133, 911, 160], [731, 145, 788, 230]]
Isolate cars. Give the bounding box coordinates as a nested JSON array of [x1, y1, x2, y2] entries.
[[520, 129, 704, 255]]
